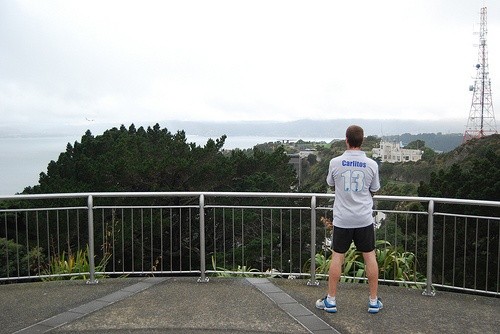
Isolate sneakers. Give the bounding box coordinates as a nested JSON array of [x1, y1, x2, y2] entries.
[[316, 297, 337, 313], [368, 300, 383, 313]]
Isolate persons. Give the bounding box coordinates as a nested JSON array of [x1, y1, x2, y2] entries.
[[316, 125, 383, 314]]
